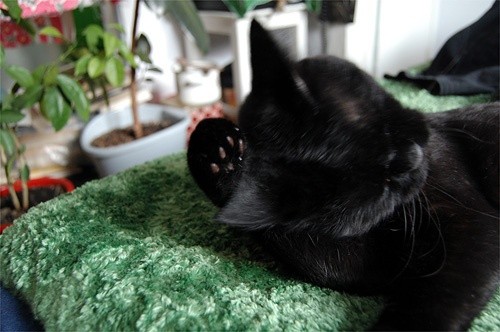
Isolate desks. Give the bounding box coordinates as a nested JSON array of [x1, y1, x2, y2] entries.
[[180, 3, 307, 118]]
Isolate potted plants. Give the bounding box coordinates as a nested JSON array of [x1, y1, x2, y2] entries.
[[79, 0, 269, 180], [0, 0, 150, 237]]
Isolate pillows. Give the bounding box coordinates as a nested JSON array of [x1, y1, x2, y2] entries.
[[0, 79, 499, 332]]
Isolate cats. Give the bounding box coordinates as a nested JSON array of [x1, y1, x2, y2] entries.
[[188, 16, 500, 331]]
